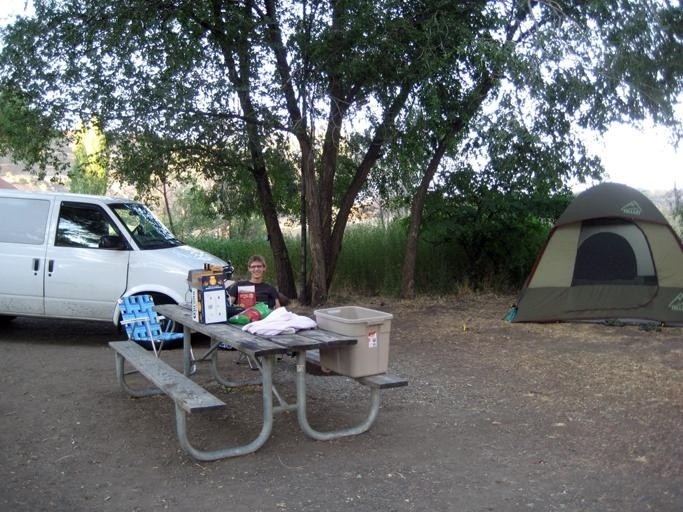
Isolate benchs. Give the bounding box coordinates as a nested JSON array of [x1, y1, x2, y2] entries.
[[293, 342, 408, 444], [109, 342, 275, 460]]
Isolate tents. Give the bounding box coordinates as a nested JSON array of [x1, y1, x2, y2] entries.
[[498, 181, 681, 330]]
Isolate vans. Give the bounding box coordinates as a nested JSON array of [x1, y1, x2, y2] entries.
[[1, 186, 236, 352]]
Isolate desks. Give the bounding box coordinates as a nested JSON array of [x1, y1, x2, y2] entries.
[[151, 301, 358, 453]]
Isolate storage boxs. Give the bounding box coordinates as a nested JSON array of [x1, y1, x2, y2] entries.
[[312, 305, 393, 379]]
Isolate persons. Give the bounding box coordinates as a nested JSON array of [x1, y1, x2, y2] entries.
[[224, 253, 283, 312]]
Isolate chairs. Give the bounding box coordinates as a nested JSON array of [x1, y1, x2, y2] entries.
[[117, 294, 196, 376]]
